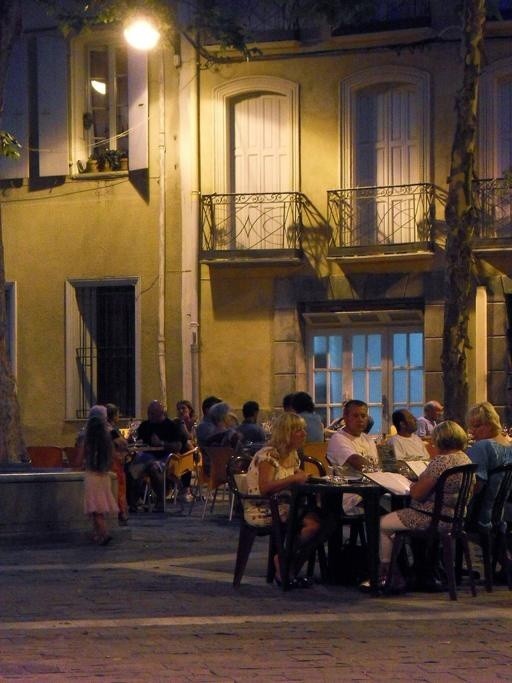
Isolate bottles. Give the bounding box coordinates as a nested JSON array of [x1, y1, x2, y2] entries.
[[376, 431, 388, 446], [190, 421, 198, 443]]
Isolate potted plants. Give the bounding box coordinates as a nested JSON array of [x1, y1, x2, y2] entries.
[[86, 153, 100, 172], [119, 150, 129, 170], [103, 148, 116, 170]]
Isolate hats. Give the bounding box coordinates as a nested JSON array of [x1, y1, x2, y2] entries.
[[209, 403, 229, 424], [88, 405, 108, 420]]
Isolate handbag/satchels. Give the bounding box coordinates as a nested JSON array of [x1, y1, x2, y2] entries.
[[341, 539, 363, 561]]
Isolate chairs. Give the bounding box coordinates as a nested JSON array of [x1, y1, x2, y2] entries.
[[234, 410, 512, 600], [24, 416, 234, 521]]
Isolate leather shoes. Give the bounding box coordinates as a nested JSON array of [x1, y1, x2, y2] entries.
[[275, 573, 314, 590]]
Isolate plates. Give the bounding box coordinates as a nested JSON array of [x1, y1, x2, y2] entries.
[[313, 473, 361, 483]]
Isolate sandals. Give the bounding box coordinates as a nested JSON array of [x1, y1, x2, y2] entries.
[[128, 502, 145, 514], [92, 532, 112, 547]]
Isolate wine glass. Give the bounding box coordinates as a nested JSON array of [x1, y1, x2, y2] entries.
[[501, 422, 512, 437], [131, 426, 141, 448], [324, 458, 413, 484]]
